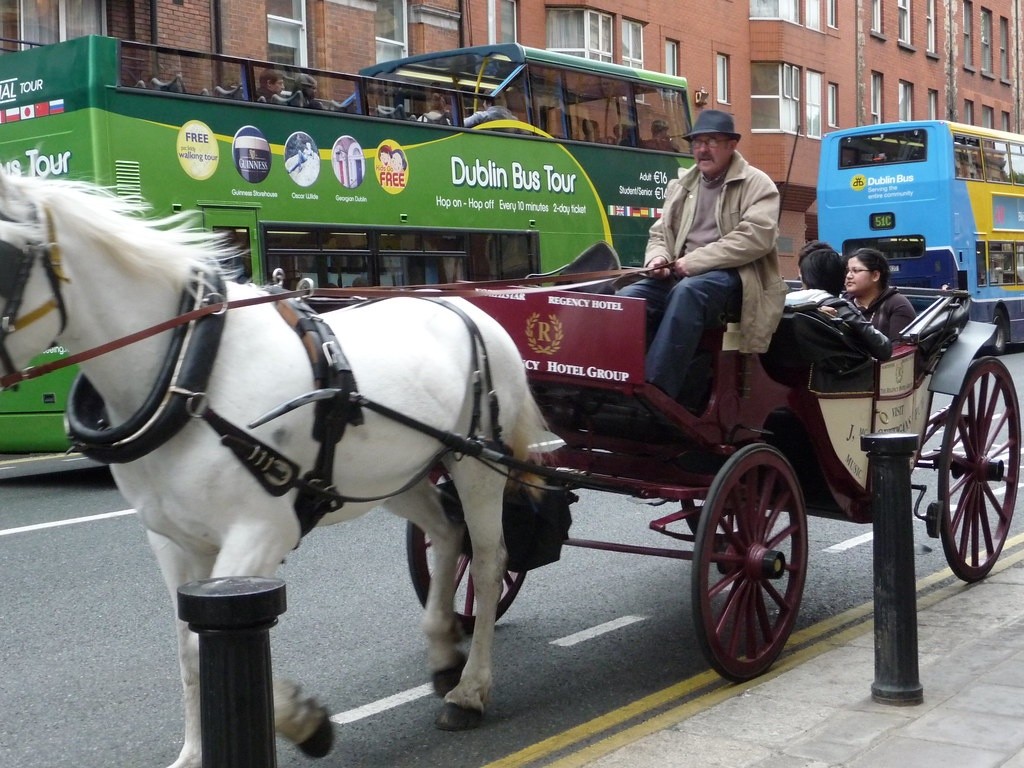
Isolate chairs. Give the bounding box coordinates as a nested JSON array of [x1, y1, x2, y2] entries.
[[134, 76, 453, 126]]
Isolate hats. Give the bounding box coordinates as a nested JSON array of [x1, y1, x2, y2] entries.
[[484, 90, 492, 95], [651, 120, 668, 130], [682, 110, 741, 140], [296, 75, 317, 86]]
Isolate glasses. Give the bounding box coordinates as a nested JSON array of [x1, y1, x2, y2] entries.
[[690, 137, 728, 147], [844, 266, 874, 273]]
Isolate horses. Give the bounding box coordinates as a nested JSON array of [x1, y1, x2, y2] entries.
[[0, 162, 557, 768]]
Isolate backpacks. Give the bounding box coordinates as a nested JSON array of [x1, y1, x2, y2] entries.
[[422, 112, 448, 125]]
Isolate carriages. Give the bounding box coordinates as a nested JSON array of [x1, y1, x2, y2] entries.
[[0, 171, 1024, 768]]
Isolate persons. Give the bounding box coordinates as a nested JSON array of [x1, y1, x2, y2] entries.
[[291, 74, 322, 108], [417, 93, 453, 127], [614, 110, 786, 398], [783, 241, 892, 361], [650, 118, 681, 153], [582, 119, 608, 144], [462, 89, 532, 136], [839, 247, 916, 345], [257, 68, 285, 103]]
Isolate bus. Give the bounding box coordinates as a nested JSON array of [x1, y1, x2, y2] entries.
[[814, 119, 1023, 354], [0, 37, 699, 460]]
